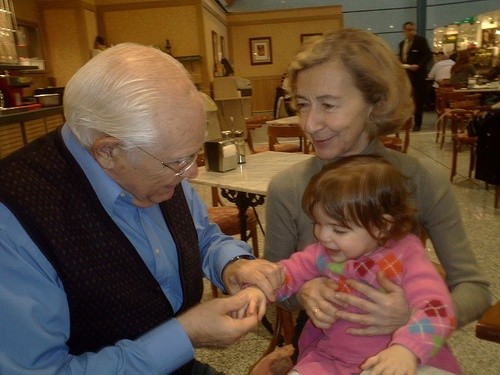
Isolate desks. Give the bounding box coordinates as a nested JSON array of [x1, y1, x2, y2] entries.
[[266, 115, 311, 153], [436, 86, 500, 182], [183, 151, 314, 347]]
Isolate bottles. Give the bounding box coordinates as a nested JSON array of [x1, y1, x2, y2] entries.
[[221, 130, 233, 140], [234, 130, 247, 164]]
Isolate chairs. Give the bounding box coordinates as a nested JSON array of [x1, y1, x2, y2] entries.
[[177, 78, 500, 375]]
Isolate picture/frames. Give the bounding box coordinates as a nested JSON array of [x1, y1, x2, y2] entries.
[[249, 37, 272, 65], [301, 33, 323, 43]]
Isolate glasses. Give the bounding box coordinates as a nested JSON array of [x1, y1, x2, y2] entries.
[[131, 146, 197, 175]]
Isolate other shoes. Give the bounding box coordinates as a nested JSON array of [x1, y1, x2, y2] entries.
[[412, 125, 420, 131]]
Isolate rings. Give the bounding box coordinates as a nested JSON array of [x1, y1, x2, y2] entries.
[[312, 309, 321, 315]]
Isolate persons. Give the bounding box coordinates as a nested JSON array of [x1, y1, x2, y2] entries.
[[398, 23, 500, 132], [0, 43, 286, 375], [227, 153, 461, 375], [263, 29, 491, 366]]
